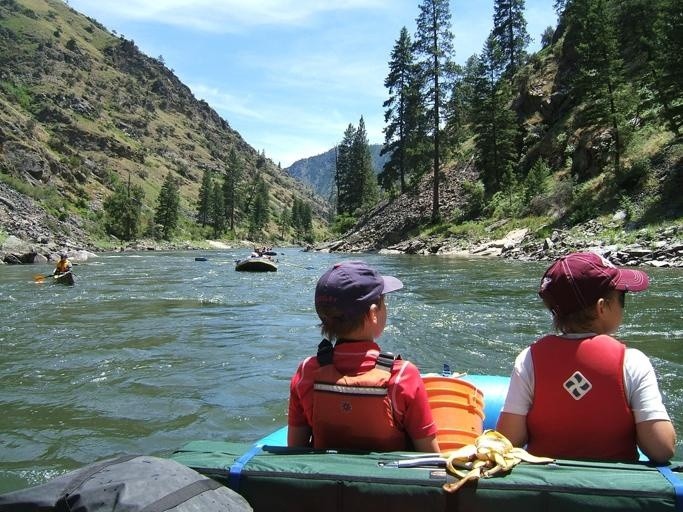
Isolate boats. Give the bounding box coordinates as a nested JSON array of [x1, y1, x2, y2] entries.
[[51, 266, 73, 286], [235, 250, 277, 272]]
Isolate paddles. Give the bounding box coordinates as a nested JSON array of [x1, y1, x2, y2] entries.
[[33, 274, 55, 281], [278, 261, 313, 270], [259, 252, 276, 255], [195, 258, 242, 262]]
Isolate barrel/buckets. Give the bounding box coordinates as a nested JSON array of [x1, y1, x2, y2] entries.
[[423, 376, 484, 454]]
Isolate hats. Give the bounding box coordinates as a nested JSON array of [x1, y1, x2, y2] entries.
[[538, 252, 649, 316], [315, 260, 404, 314]]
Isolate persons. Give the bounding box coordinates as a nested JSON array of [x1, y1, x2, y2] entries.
[[287, 262, 441, 452], [494, 253, 676, 463], [56, 254, 73, 272]]
